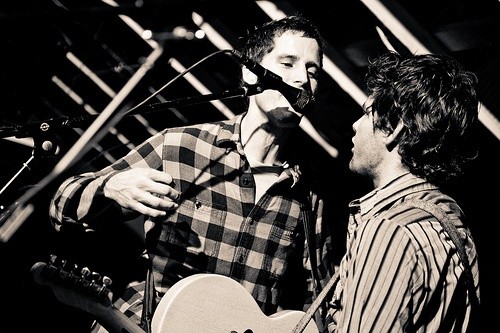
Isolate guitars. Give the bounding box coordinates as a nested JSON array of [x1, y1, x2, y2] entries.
[[28, 254, 321, 329]]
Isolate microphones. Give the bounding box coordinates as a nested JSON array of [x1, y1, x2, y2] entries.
[[231, 49, 316, 114]]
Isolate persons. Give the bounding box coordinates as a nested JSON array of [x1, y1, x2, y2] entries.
[[49, 14, 334, 333], [327, 50, 481, 332]]
